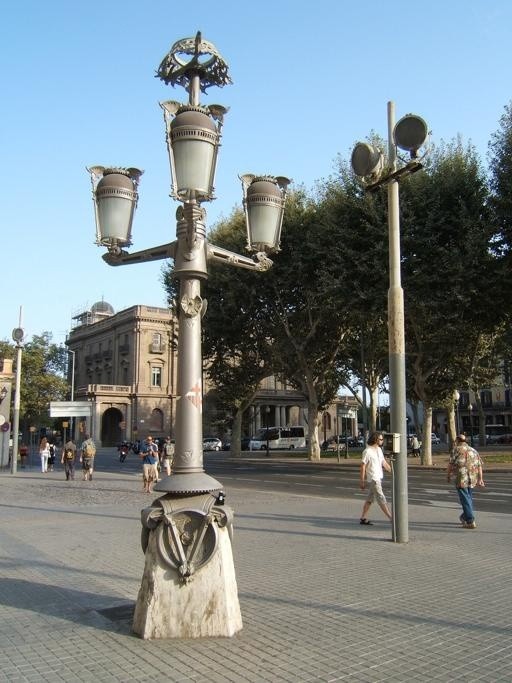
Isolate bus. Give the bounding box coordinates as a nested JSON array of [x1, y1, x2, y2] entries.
[[464, 423, 512, 444]]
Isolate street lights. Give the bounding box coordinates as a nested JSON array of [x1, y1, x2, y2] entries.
[[9, 304, 26, 473], [454, 389, 459, 436], [65, 346, 77, 442], [344, 398, 351, 459], [349, 97, 432, 541], [87, 29, 292, 637], [265, 403, 271, 458], [467, 402, 473, 434]]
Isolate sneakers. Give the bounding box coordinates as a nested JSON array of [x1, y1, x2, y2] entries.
[[460, 516, 476, 529]]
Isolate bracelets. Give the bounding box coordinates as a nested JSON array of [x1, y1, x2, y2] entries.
[[447, 475, 451, 479]]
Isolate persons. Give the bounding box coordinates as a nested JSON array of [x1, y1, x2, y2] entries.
[[18, 439, 31, 468], [139, 435, 160, 493], [47, 438, 58, 471], [445, 434, 485, 528], [61, 436, 78, 480], [39, 435, 50, 472], [160, 435, 175, 475], [359, 431, 391, 525], [79, 433, 97, 480], [410, 434, 420, 457]]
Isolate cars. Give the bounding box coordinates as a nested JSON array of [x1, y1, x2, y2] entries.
[[132, 435, 164, 454], [203, 424, 440, 453]]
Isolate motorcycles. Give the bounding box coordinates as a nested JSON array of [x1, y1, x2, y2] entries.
[[118, 440, 130, 461]]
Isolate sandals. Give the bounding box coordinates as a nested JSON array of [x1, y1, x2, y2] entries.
[[360, 517, 372, 525]]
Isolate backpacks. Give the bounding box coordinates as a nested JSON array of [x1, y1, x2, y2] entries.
[[51, 445, 55, 455], [84, 441, 95, 458], [64, 443, 74, 459], [165, 443, 174, 456]]
